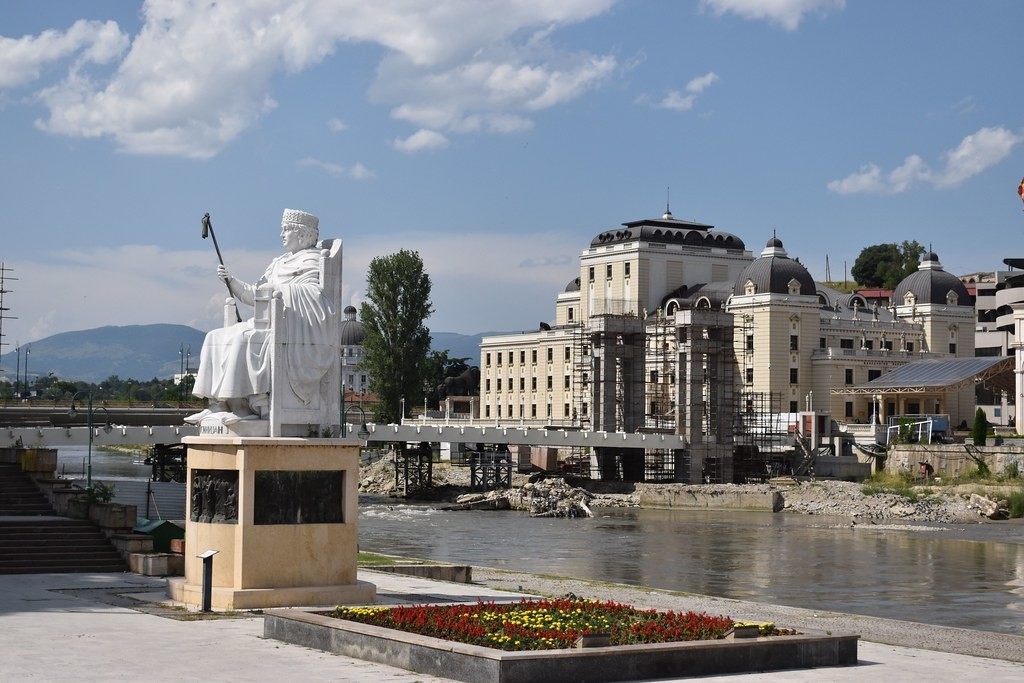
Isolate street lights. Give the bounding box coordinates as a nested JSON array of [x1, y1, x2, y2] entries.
[[424, 397, 427, 418], [872, 395, 876, 425], [341, 383, 370, 440], [25, 348, 30, 404], [128, 380, 132, 408], [68, 389, 113, 496], [402, 398, 405, 419], [179, 342, 191, 401], [469, 396, 474, 419], [445, 398, 450, 419], [14, 347, 20, 405]]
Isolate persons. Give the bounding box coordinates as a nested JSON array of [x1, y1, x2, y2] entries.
[[184, 208, 330, 426]]
